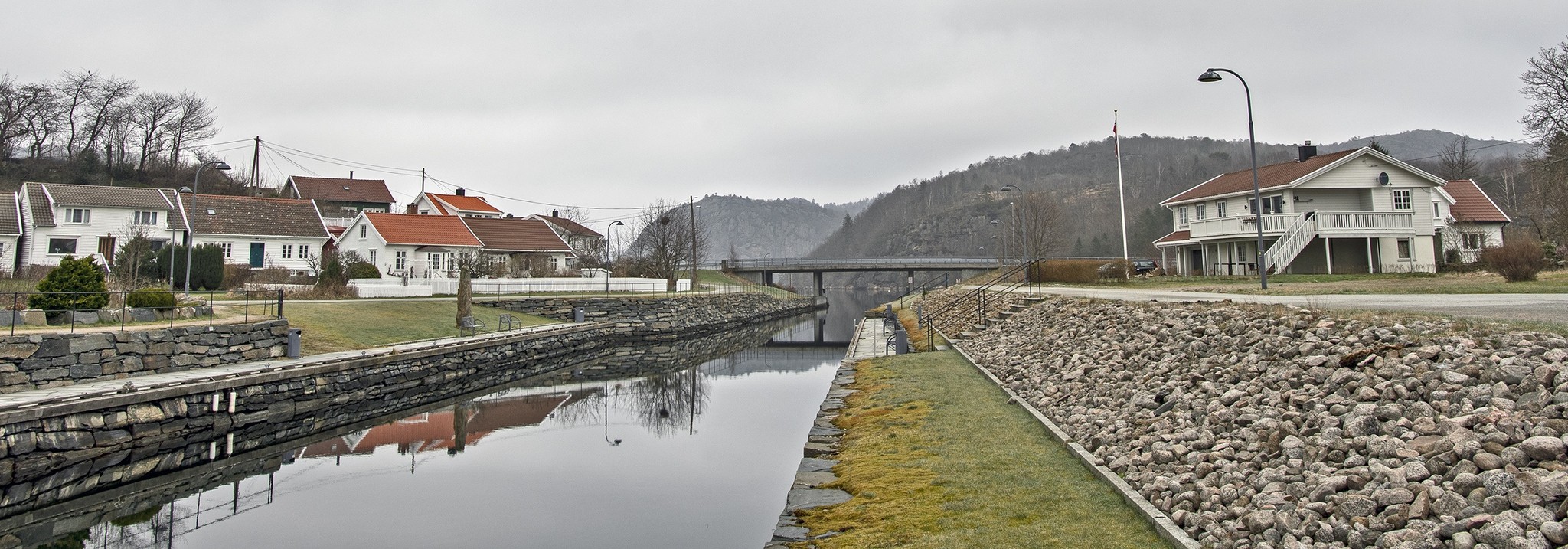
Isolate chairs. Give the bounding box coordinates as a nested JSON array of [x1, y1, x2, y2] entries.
[[1248, 263, 1260, 276]]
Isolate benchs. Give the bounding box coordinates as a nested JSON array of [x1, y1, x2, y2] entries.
[[498, 313, 521, 332], [460, 316, 486, 337]]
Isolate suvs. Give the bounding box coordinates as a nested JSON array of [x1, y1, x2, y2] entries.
[[1098, 258, 1155, 277]]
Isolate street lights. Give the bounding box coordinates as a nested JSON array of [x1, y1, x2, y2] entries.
[[990, 219, 1008, 284], [979, 246, 989, 273], [696, 206, 701, 266], [1196, 69, 1269, 290], [1000, 185, 1028, 286], [606, 221, 624, 293], [1010, 202, 1016, 266], [764, 254, 770, 286], [690, 196, 698, 280], [605, 380, 622, 445], [185, 161, 232, 296], [169, 186, 193, 294]]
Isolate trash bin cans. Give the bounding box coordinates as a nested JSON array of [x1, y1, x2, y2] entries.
[[286, 329, 302, 358], [574, 307, 585, 323]]
